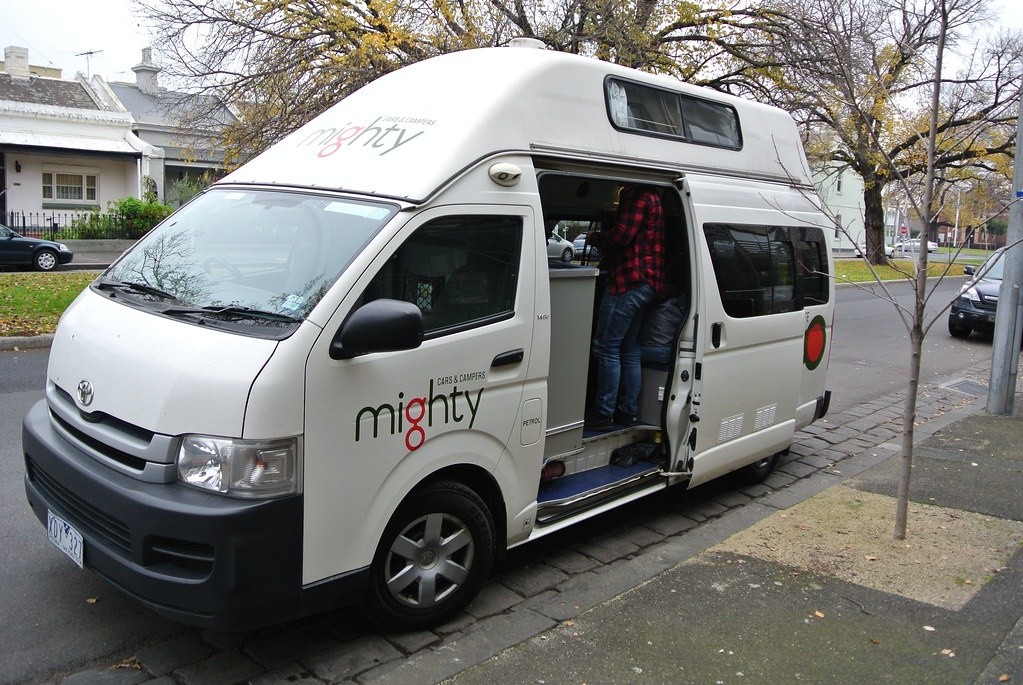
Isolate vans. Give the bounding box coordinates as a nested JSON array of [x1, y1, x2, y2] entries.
[[19, 37, 833, 635]]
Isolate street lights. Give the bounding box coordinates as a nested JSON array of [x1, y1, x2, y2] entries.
[[953, 186, 974, 247]]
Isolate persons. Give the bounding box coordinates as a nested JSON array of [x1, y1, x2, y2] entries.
[[584, 181, 689, 429]]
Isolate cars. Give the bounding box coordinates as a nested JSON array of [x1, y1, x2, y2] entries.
[[547, 231, 575, 262], [572, 232, 597, 257], [854, 242, 895, 258], [0, 224, 73, 271], [948, 244, 1005, 335], [893, 238, 938, 253]]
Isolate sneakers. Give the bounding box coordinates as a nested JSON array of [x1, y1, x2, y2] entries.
[[615, 409, 638, 426], [584, 409, 613, 430]]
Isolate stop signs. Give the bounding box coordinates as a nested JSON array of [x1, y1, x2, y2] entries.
[[900, 227, 908, 234]]
